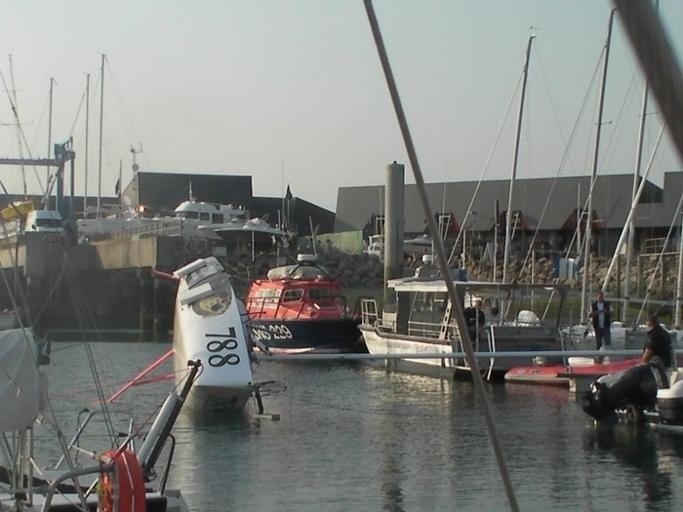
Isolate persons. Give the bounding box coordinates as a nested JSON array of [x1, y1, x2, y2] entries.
[[462, 300, 484, 343], [640, 313, 672, 389], [586, 291, 613, 350]]
